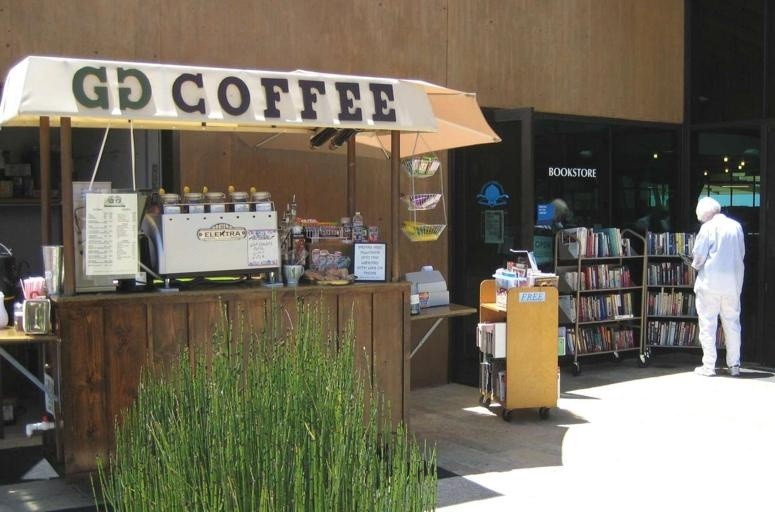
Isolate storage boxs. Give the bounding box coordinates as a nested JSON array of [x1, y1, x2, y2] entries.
[[492, 273, 527, 312]]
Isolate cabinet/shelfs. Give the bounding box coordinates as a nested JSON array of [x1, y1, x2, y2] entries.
[[0, 324, 63, 482], [476, 277, 559, 421], [626, 226, 727, 365], [553, 227, 648, 374]]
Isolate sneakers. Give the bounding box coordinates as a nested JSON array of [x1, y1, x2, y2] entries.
[[695, 365, 716, 376], [727, 365, 740, 378]]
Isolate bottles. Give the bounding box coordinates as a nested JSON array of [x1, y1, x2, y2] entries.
[[284, 194, 297, 225], [13, 302, 28, 331], [408, 279, 421, 316], [341, 211, 365, 242]]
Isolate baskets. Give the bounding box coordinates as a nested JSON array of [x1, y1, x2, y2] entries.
[[400, 158, 447, 242]]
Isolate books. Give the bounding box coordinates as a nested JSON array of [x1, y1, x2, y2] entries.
[[559, 228, 725, 356], [475, 247, 562, 401]]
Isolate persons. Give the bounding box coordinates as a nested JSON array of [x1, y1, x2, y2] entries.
[[690, 195, 745, 376]]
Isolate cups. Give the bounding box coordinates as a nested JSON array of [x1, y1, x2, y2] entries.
[[283, 264, 304, 285], [42, 245, 65, 297]]
